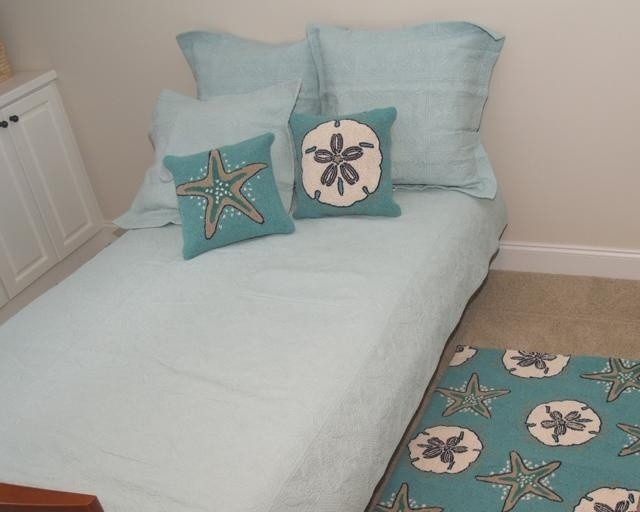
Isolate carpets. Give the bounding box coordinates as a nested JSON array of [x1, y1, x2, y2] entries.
[[368, 345, 640, 512]]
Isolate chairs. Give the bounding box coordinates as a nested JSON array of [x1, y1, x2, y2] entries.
[[0, 483, 105, 512]]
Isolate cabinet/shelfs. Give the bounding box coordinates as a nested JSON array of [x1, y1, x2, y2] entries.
[[0, 65, 104, 307]]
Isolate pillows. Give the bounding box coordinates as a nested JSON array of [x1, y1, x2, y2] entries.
[[287, 104, 401, 221], [111, 78, 302, 231], [306, 22, 503, 199], [177, 32, 320, 134], [160, 132, 294, 260]]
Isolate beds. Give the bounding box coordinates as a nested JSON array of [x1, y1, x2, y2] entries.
[[0, 180, 506, 512]]
